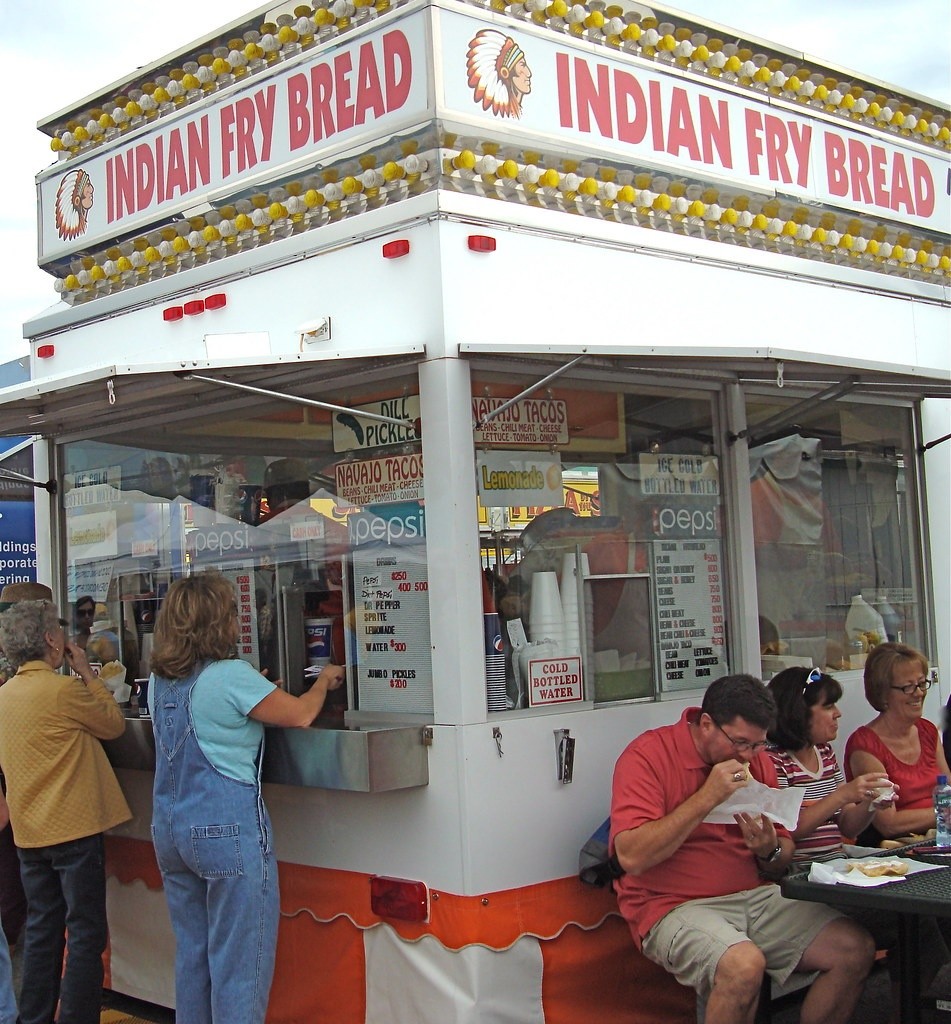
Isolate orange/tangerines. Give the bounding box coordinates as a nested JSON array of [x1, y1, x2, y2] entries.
[[848, 631, 880, 655]]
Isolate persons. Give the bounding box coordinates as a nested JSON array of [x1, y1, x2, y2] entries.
[[609, 672, 876, 1024], [315, 543, 382, 696], [0, 599, 133, 1024], [72, 595, 122, 667], [579, 472, 658, 662], [0, 582, 71, 958], [752, 464, 894, 641], [762, 666, 951, 1024], [146, 570, 343, 1024], [844, 642, 951, 847], [0, 781, 22, 1023]]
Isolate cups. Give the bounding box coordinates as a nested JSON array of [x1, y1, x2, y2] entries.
[[529, 552, 594, 659], [484, 612, 507, 712], [135, 591, 155, 678], [303, 617, 334, 669], [190, 473, 263, 526]]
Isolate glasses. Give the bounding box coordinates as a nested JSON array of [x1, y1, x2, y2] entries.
[[76, 609, 94, 618], [891, 679, 932, 695], [801, 666, 823, 696], [705, 711, 767, 752]]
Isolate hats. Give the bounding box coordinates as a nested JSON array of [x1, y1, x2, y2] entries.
[[0, 583, 68, 627]]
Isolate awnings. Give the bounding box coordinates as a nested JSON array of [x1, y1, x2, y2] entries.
[[455, 339, 951, 456], [0, 343, 427, 503]]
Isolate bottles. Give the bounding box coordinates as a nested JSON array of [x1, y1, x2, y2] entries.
[[932, 775, 951, 847], [845, 595, 904, 652]]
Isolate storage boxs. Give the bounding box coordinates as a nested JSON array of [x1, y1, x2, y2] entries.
[[583, 668, 653, 702], [826, 638, 868, 671]]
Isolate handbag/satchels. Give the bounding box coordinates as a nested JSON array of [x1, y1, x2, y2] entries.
[[579, 813, 624, 888]]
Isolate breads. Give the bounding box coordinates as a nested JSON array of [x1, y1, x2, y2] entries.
[[98, 661, 124, 681], [846, 860, 908, 878]]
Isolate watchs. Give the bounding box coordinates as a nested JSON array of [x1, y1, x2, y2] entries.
[[757, 843, 782, 863]]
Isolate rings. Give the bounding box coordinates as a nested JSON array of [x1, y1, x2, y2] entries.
[[866, 790, 874, 797], [734, 772, 742, 781]]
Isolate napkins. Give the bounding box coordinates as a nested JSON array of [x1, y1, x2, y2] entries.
[[809, 861, 838, 886], [848, 854, 950, 876], [807, 858, 907, 888]]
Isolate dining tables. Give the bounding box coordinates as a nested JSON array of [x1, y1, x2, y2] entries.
[[781, 838, 951, 1024]]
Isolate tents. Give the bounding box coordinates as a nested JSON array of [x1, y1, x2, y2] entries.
[[69, 487, 426, 702]]
